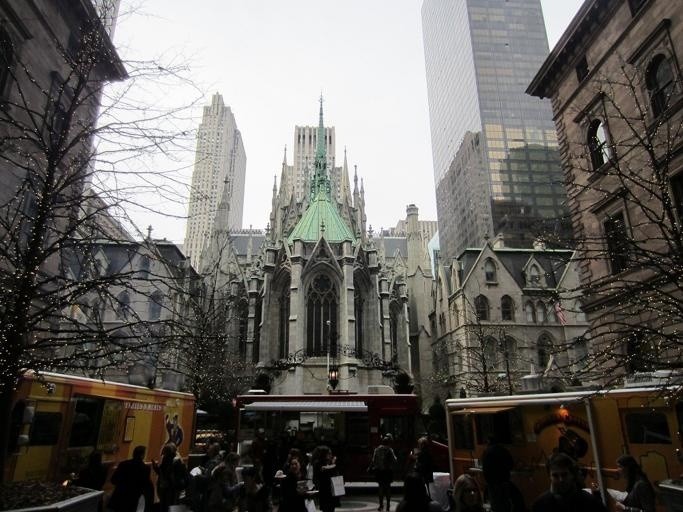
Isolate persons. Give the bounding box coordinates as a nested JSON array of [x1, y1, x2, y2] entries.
[[448, 473, 485, 511], [528, 454, 614, 511], [165, 413, 183, 449], [113, 427, 345, 511], [576, 467, 587, 487], [396, 475, 442, 511], [370, 432, 399, 511], [602, 455, 656, 512], [480, 430, 530, 511], [409, 437, 431, 494]]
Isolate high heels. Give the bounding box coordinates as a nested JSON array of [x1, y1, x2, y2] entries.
[[378, 504, 384, 511]]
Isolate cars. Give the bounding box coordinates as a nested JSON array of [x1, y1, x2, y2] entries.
[[194, 428, 226, 448]]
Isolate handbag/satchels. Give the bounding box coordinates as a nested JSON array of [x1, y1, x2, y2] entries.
[[330, 475, 345, 497], [366, 458, 380, 473], [405, 450, 421, 484]]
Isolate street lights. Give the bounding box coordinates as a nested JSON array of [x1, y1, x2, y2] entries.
[[327, 367, 340, 390]]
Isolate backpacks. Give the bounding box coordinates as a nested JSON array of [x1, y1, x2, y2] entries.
[[189, 463, 226, 511]]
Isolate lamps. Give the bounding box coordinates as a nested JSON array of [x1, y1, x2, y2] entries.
[[327, 362, 340, 390]]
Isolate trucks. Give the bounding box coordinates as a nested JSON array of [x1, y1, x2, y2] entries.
[[0, 363, 200, 496], [440, 383, 682, 497], [229, 385, 430, 488]]
